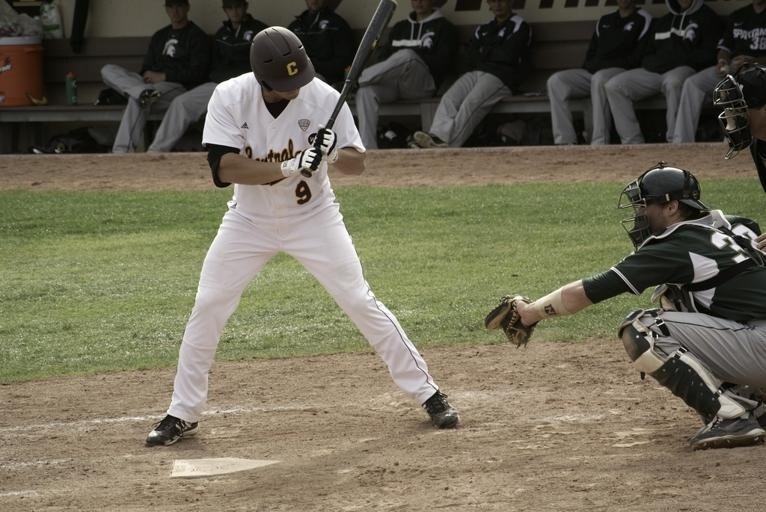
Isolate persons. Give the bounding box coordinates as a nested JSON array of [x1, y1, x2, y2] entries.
[[485, 163, 766, 449], [143, 26, 459, 448], [98, 2, 766, 194]]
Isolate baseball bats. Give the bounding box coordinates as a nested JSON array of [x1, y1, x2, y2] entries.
[[300, 0, 397, 178]]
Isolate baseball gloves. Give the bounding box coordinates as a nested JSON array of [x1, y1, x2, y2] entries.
[[485, 296, 538, 350]]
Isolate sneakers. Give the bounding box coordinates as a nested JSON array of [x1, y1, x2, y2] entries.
[[407, 131, 448, 148], [690, 396, 766, 450], [421, 390, 458, 428], [146, 415, 199, 445]]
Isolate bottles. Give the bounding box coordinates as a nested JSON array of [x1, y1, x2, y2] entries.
[[62, 64, 78, 105]]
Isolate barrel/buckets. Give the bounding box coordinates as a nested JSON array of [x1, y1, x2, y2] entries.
[[40, 4, 63, 39], [0, 36, 48, 107]]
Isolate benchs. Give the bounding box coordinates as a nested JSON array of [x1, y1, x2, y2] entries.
[[0, 19, 712, 152]]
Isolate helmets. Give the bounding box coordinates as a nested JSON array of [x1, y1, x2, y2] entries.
[[250, 26, 315, 92], [618, 161, 711, 253], [713, 62, 766, 160]]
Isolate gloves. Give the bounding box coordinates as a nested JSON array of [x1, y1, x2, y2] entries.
[[280, 147, 324, 177], [310, 124, 338, 165]]
